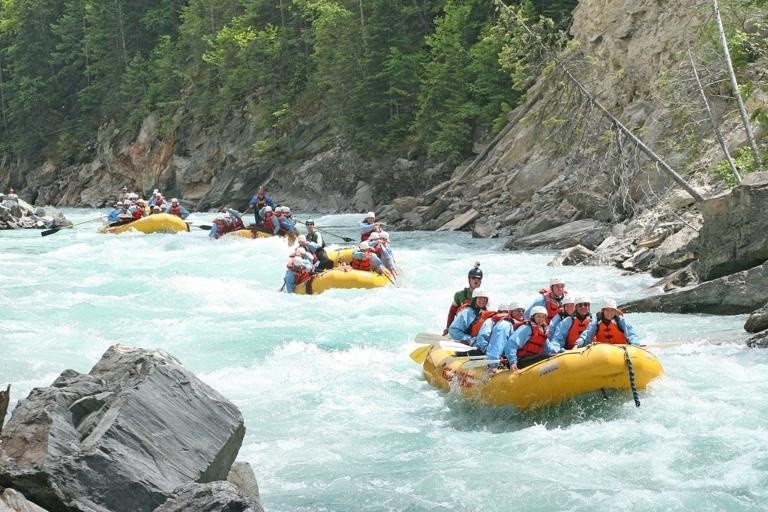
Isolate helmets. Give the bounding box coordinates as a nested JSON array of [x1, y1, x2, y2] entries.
[[472, 288, 488, 298], [116, 189, 177, 210], [468, 268, 483, 279], [264, 205, 290, 212], [550, 275, 565, 286], [498, 302, 548, 318], [561, 296, 617, 309], [293, 234, 306, 266], [359, 231, 389, 250], [305, 219, 314, 226], [366, 212, 375, 218]]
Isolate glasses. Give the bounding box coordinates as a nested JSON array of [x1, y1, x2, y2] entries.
[[471, 277, 480, 281], [306, 223, 312, 225]]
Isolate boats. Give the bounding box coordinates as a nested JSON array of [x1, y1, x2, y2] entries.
[[294, 249, 389, 295], [219, 223, 299, 240], [423, 332, 663, 414], [97, 213, 190, 235]]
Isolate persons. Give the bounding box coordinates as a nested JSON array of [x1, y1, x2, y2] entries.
[[442, 261, 641, 375], [288, 211, 395, 279], [209, 185, 293, 239], [107, 186, 189, 230]]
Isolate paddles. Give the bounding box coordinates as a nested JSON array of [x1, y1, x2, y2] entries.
[[411, 334, 444, 363], [187, 225, 212, 231], [42, 215, 108, 236], [295, 218, 354, 243]]
